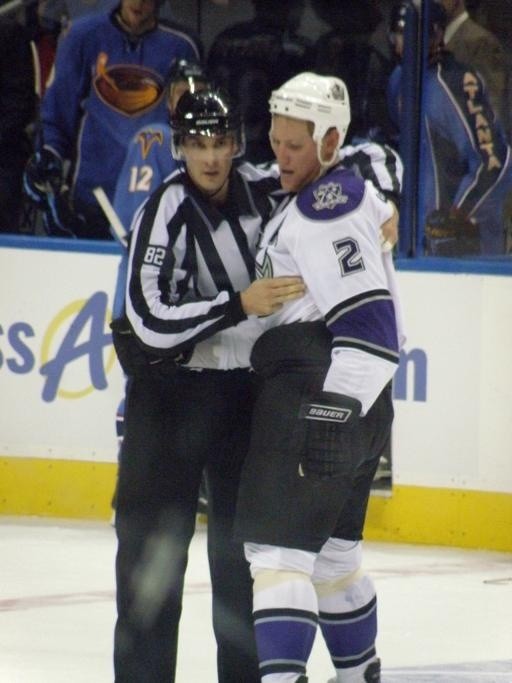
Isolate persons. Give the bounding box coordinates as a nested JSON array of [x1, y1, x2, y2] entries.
[[110, 86, 396, 682], [236, 71, 399, 682], [0, 0, 511, 273]]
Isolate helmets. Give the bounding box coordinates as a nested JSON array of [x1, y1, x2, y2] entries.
[[167, 81, 246, 162], [268, 71, 352, 146]]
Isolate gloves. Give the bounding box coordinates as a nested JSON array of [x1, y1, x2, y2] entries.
[[22, 148, 69, 211]]
